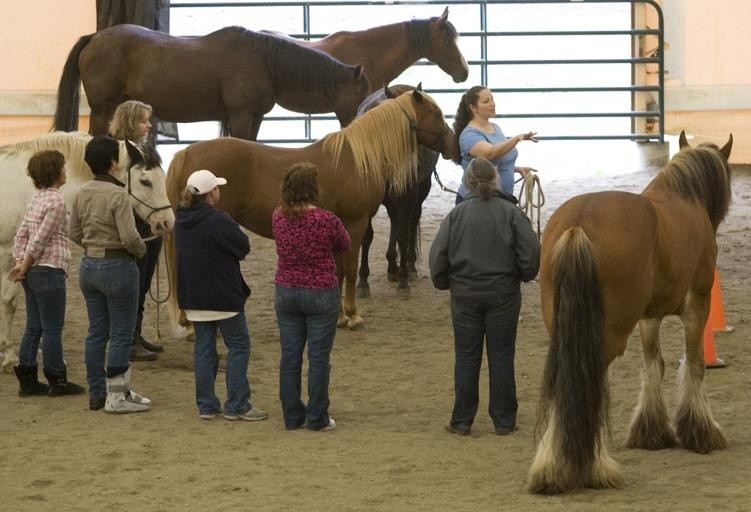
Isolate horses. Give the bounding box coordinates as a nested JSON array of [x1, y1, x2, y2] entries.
[[47, 23, 369, 142], [162, 89, 459, 343], [353, 81, 440, 301], [219, 5, 470, 138], [0, 130, 176, 375], [524, 129, 734, 496]]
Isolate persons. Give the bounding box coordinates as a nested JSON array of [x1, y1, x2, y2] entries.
[[272, 163, 352, 430], [69, 135, 152, 414], [8, 150, 86, 398], [107, 100, 167, 363], [173, 169, 269, 422], [450, 85, 539, 212], [428, 157, 541, 436]]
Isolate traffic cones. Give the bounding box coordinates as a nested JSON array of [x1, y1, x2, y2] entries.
[[677, 320, 728, 371], [705, 268, 734, 334]]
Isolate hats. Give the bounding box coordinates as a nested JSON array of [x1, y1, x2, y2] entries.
[[187, 170, 227, 195]]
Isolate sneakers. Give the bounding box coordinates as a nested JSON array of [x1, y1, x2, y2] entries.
[[322, 417, 336, 431], [200, 410, 222, 419], [224, 406, 268, 421], [130, 342, 158, 361], [142, 338, 164, 353], [444, 425, 468, 434], [495, 423, 519, 434]]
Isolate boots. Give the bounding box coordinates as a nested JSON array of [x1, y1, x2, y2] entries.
[[13, 362, 49, 397], [43, 366, 85, 397], [103, 365, 151, 413]]
